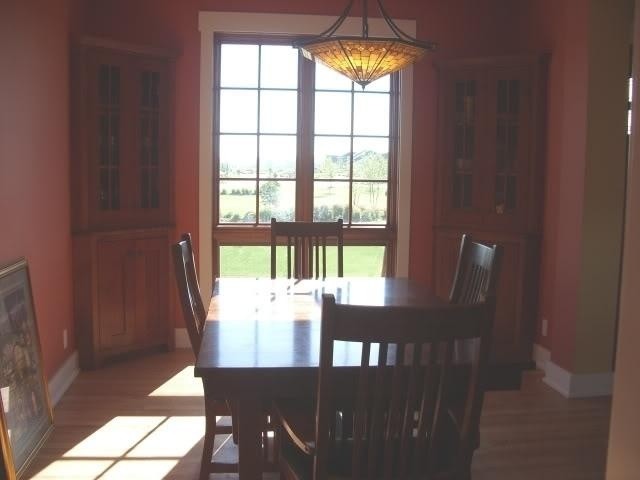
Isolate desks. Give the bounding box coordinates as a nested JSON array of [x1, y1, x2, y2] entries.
[[193, 278, 474, 479]]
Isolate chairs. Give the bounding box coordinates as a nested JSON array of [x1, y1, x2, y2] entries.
[[265, 293, 491, 479], [448, 235, 500, 320], [175, 232, 274, 480], [269, 218, 344, 278]]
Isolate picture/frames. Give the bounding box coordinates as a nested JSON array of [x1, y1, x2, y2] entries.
[[0, 252, 54, 480]]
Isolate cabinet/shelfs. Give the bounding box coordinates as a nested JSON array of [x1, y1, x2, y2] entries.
[[433, 50, 545, 372], [68, 28, 177, 375]]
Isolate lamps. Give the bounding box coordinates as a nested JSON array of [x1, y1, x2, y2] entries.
[[290, 0, 438, 94]]
